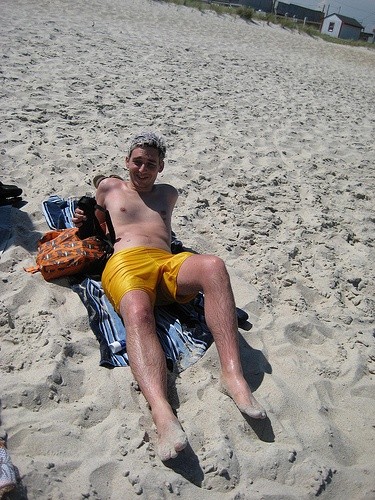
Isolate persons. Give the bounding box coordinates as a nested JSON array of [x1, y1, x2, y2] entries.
[[72, 132, 267, 460]]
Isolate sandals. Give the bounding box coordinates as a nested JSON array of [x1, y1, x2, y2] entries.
[[94, 175, 125, 188]]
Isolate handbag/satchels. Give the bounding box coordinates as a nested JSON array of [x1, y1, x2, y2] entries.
[[23, 228, 107, 281]]
